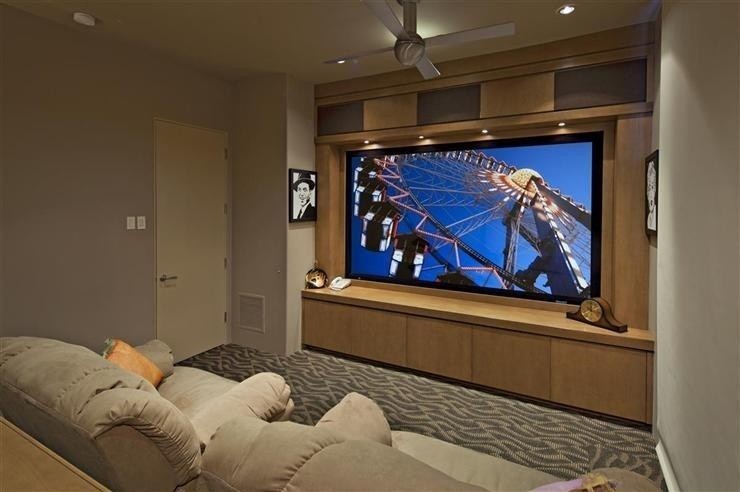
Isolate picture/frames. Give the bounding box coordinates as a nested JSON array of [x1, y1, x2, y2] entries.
[[644, 148, 660, 236], [288, 167, 318, 223]]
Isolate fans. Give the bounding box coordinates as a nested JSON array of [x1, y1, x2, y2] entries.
[[320, 0, 516, 81]]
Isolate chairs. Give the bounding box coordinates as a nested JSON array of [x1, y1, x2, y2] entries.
[[201, 391, 659, 491], [0, 337, 294, 491]]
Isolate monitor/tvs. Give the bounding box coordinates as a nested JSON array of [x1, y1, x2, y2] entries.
[[346, 129, 606, 305]]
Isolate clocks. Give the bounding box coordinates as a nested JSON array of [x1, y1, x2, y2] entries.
[[566, 297, 627, 334]]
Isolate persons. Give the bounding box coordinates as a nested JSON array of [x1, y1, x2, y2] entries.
[[292, 170, 317, 221]]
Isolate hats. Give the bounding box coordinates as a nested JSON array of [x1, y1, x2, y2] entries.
[[293, 173, 315, 191]]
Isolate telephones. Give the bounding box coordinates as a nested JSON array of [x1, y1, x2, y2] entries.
[[328, 276, 352, 291]]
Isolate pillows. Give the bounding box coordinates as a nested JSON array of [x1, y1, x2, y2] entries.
[[100, 338, 164, 388]]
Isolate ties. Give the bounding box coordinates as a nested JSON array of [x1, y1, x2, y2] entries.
[[297, 209, 302, 218]]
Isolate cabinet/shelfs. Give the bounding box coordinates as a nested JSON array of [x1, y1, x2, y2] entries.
[[299, 281, 655, 429]]
